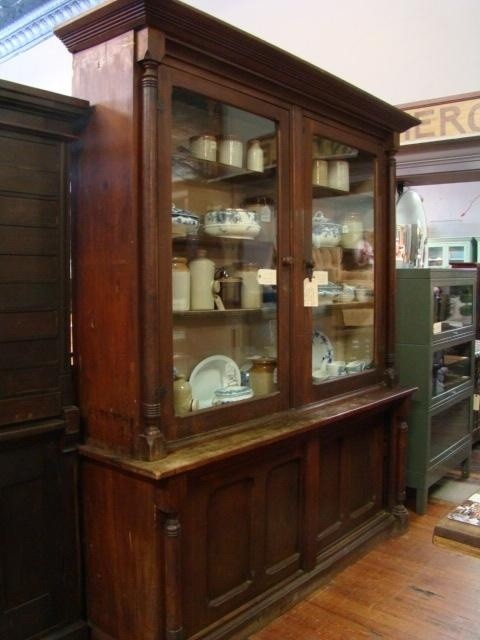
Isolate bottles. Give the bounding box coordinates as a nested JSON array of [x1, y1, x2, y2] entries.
[[171, 250, 263, 312], [342, 211, 363, 249], [249, 358, 281, 397], [248, 139, 264, 172], [172, 373, 192, 416]]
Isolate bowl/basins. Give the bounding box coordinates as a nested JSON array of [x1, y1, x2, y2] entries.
[[309, 222, 342, 247], [318, 287, 342, 304], [188, 355, 241, 409], [172, 208, 260, 238]]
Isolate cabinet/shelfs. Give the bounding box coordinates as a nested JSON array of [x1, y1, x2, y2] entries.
[[52, 1, 420, 640], [389, 237, 478, 516]]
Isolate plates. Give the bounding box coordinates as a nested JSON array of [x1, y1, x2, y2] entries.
[[312, 329, 333, 379]]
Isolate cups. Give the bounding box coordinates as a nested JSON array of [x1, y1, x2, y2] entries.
[[311, 159, 350, 193], [189, 132, 243, 169], [355, 289, 372, 302]]
